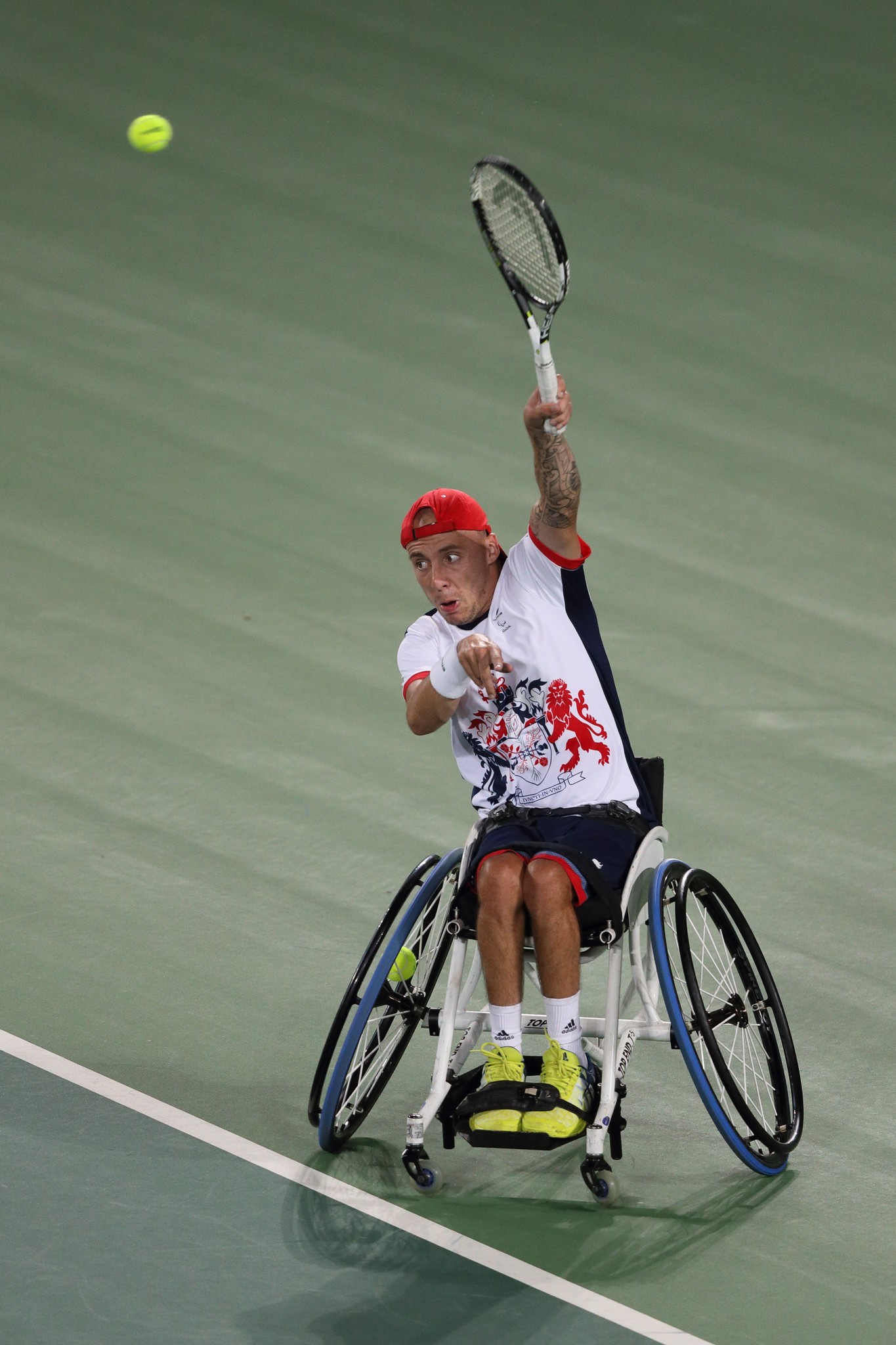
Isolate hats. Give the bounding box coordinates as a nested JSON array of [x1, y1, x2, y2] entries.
[[400, 487, 508, 559]]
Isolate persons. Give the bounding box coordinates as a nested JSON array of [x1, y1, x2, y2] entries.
[[395, 371, 645, 1138]]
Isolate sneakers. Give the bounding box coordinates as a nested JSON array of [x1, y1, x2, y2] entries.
[[469, 1042, 527, 1133], [522, 1026, 598, 1139]]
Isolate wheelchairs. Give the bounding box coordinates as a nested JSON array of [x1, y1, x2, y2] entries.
[[306, 753, 804, 1200]]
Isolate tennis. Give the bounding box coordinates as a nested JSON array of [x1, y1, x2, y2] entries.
[[127, 113, 172, 154]]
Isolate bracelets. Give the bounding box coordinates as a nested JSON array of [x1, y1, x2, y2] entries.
[[429, 641, 472, 700]]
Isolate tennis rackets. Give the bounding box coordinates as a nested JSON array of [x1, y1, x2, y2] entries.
[[468, 152, 574, 436]]
